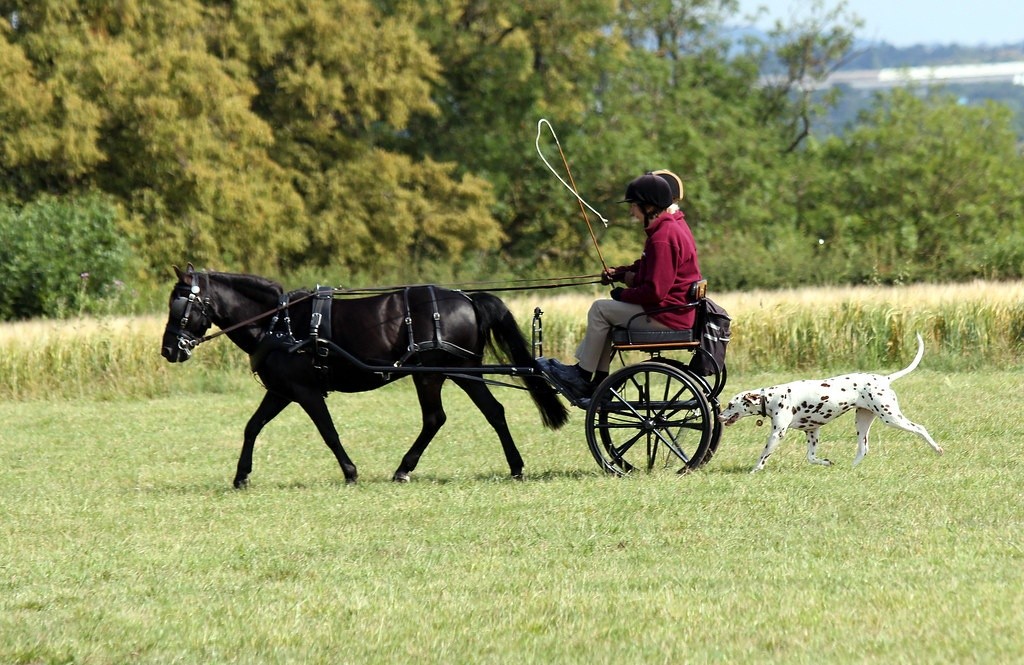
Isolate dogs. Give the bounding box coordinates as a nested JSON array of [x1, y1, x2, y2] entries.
[[717, 332, 944, 474]]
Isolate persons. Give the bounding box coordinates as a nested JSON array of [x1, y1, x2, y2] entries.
[[597, 168, 696, 286], [546, 174, 701, 395]]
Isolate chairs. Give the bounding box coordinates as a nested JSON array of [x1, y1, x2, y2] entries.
[[610, 278, 709, 358]]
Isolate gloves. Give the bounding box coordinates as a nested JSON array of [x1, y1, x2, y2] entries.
[[610, 287, 625, 301]]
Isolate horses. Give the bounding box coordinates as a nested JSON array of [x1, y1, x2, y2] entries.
[[160, 262, 572, 490]]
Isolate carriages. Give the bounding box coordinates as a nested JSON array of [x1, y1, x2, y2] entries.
[[158, 258, 732, 487]]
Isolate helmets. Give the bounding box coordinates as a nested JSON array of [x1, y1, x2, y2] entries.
[[625, 173, 673, 208], [648, 167, 684, 202]]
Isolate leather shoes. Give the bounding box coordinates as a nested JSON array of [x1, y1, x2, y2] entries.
[[582, 370, 610, 398], [549, 358, 594, 386], [548, 365, 592, 400]]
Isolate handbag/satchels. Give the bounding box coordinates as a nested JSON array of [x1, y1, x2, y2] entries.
[[691, 298, 732, 377]]
